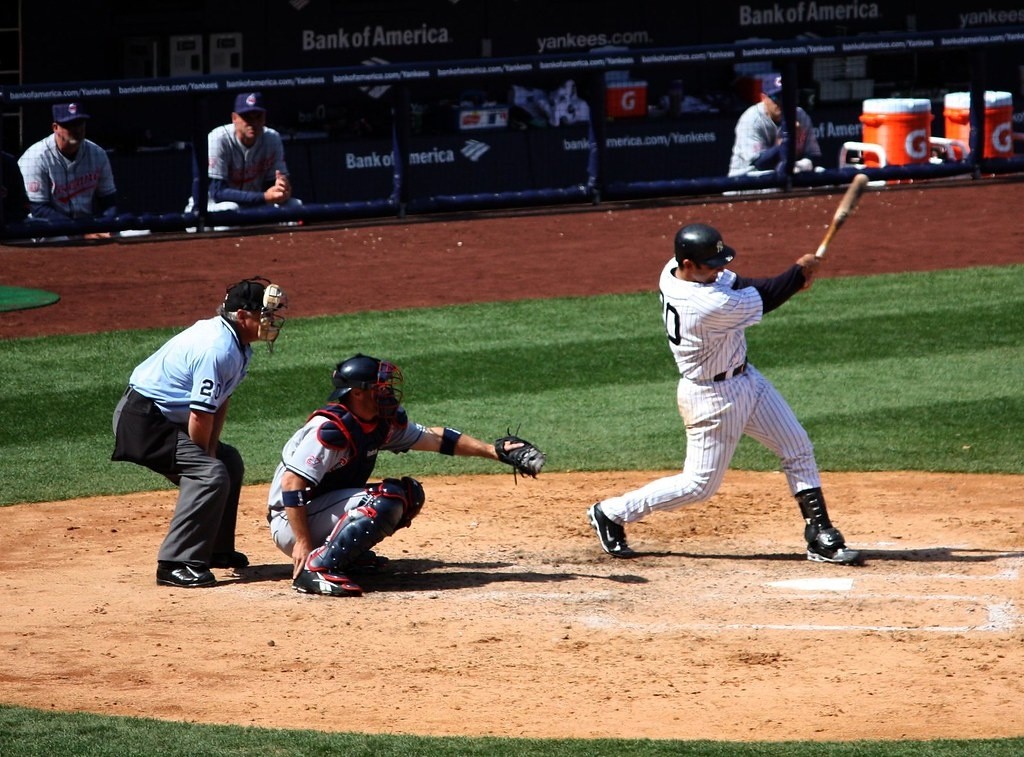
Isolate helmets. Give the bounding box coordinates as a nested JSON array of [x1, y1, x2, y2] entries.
[[674, 224, 736, 267], [327, 357, 385, 401]]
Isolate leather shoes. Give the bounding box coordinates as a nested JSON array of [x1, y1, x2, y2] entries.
[[217, 550, 251, 569], [156, 565, 218, 588]]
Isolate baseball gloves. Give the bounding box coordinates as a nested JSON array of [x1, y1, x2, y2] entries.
[[494, 436, 547, 476]]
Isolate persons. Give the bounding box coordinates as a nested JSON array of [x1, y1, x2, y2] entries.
[[17, 101, 150, 239], [113, 278, 285, 587], [267, 356, 546, 596], [185, 92, 302, 232], [723, 75, 823, 195], [586, 224, 862, 563]]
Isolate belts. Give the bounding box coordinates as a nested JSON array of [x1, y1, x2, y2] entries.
[[266, 511, 272, 522], [125, 387, 134, 397], [714, 357, 747, 382]]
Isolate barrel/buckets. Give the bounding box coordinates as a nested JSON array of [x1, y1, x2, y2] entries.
[[859, 97, 933, 167], [942, 90, 1013, 160]]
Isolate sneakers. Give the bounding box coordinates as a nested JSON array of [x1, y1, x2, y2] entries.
[[357, 553, 388, 570], [291, 569, 350, 596], [588, 502, 635, 557], [806, 530, 862, 566]]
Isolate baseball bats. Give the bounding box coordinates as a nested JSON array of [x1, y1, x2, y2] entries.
[[816, 173, 869, 260]]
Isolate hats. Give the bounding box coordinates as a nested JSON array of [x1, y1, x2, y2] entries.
[[234, 91, 267, 114], [762, 73, 782, 95], [52, 102, 91, 124], [225, 282, 283, 311]]
